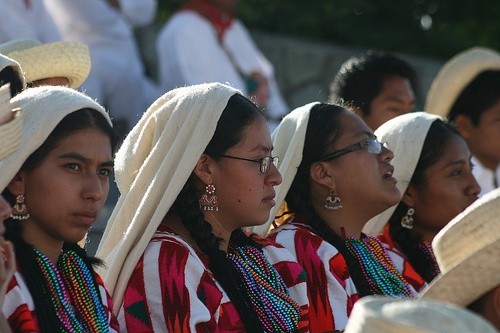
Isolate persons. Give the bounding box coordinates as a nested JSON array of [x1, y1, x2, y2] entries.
[[93, 83, 306, 333], [0, 0, 500, 237], [0, 189, 17, 333], [0, 86, 120, 333], [414, 187, 499, 331], [338, 295, 495, 332], [258, 101, 435, 333]]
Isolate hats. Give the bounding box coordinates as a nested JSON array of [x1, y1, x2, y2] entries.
[[416, 187, 500, 309], [0, 82, 25, 161], [344, 295, 498, 333], [425, 47, 500, 123], [0, 39, 92, 90]]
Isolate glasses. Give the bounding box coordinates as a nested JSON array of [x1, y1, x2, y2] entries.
[[319, 139, 390, 163], [211, 154, 280, 174]]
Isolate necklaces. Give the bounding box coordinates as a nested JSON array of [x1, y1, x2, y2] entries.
[[346, 235, 440, 301], [34, 248, 109, 333], [225, 244, 302, 333]]
